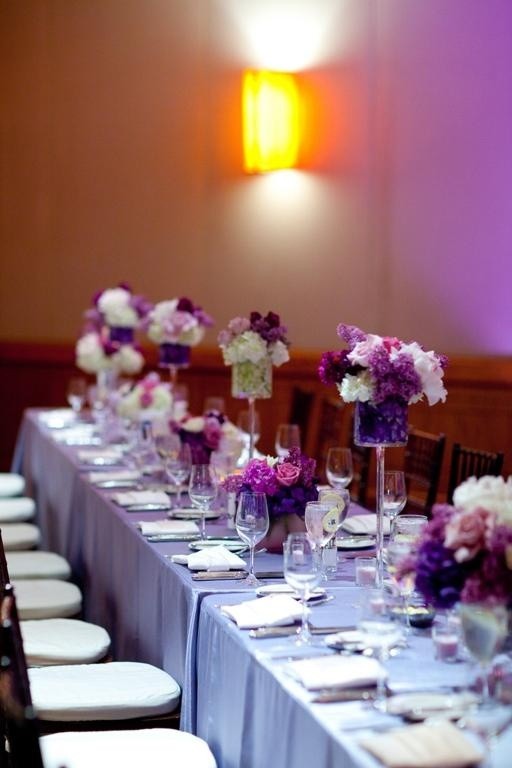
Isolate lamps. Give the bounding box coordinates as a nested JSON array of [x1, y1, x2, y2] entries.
[[242, 70, 302, 173]]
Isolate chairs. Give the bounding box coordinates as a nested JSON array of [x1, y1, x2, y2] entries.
[[1, 386, 511, 767]]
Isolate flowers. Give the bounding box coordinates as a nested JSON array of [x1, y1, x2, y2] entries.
[[146, 296, 216, 369], [75, 279, 155, 375], [316, 322, 453, 447], [213, 310, 293, 398]]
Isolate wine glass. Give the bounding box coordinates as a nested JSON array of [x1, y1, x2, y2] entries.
[[64, 358, 508, 728]]
